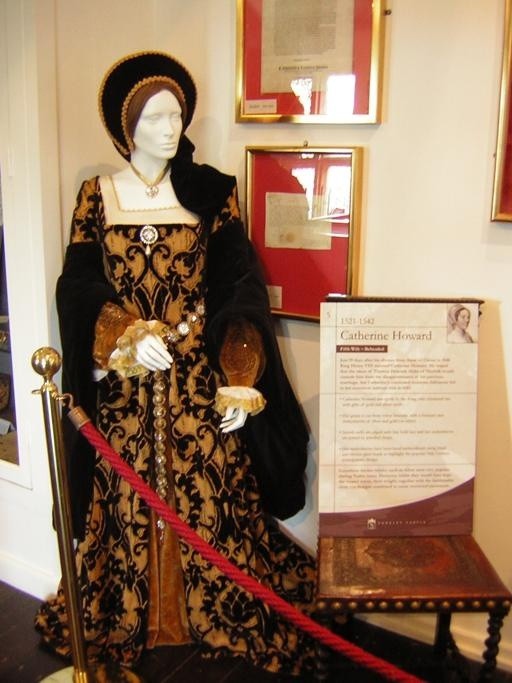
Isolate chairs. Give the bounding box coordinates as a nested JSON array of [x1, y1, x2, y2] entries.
[[315, 296, 512, 683]]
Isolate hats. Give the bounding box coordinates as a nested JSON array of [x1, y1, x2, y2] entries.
[[97, 50, 198, 155]]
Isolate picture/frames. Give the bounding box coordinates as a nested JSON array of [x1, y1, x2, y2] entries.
[[491, 0, 512, 222], [235, 0, 386, 125], [244, 145, 364, 324]]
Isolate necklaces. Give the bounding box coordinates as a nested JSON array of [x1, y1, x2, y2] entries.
[[127, 159, 174, 200]]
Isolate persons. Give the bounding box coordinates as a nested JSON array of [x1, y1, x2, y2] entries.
[[444, 299, 474, 346], [47, 50, 274, 661]]
